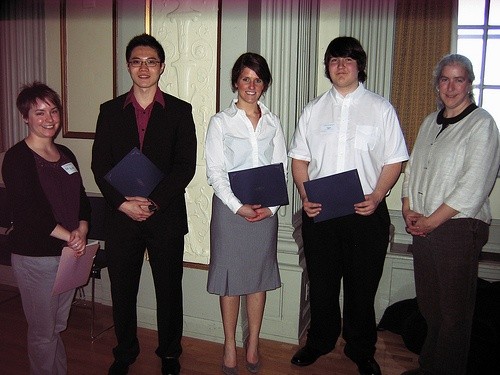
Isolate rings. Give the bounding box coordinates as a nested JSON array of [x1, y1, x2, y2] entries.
[[75, 244, 78, 246]]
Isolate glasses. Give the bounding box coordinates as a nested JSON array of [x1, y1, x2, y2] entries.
[[128, 60, 160, 67]]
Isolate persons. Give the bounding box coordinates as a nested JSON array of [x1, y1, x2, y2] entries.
[[204, 52, 287, 375], [91, 33, 197, 375], [287, 36, 408, 375], [401, 54, 500, 375], [1, 81, 90, 375]]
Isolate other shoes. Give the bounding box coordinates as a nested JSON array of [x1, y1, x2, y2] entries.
[[245, 336, 260, 373], [108, 358, 136, 375], [291, 344, 335, 366], [161, 357, 181, 375], [344, 345, 382, 375], [223, 342, 237, 375]]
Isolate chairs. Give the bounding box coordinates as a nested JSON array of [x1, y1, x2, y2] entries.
[[71, 196, 115, 344]]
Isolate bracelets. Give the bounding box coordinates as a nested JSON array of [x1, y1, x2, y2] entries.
[[147, 200, 158, 212]]
